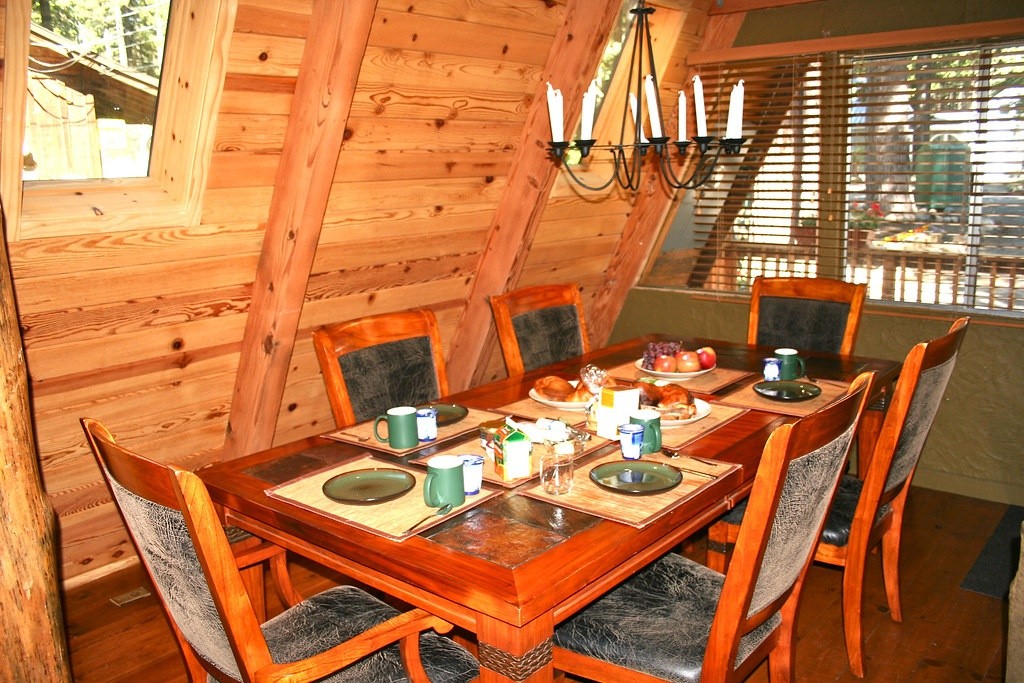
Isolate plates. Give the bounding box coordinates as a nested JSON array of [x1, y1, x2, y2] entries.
[[323, 467, 416, 505], [635, 356, 718, 381], [529, 379, 615, 410], [410, 404, 468, 426], [589, 459, 683, 495], [633, 393, 712, 429], [753, 379, 822, 401]]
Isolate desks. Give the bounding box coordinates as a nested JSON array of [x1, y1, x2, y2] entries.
[[195, 333, 902, 683]]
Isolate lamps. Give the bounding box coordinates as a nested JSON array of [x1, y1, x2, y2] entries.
[[546, 0, 750, 189]]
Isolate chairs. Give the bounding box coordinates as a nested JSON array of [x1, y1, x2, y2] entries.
[[749, 274, 868, 359], [313, 306, 451, 427], [561, 370, 875, 683], [489, 284, 591, 376], [707, 318, 969, 683], [77, 415, 483, 683]]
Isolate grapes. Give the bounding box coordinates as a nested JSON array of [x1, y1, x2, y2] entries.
[[642, 341, 683, 371]]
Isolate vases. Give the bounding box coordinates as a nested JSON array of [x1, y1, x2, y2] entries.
[[791, 224, 876, 249]]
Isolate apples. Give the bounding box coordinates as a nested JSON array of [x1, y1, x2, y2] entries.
[[654, 345, 716, 373]]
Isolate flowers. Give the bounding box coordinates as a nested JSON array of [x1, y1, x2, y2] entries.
[[801, 201, 886, 229]]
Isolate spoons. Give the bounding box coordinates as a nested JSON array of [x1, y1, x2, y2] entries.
[[402, 503, 453, 534], [660, 446, 717, 468]]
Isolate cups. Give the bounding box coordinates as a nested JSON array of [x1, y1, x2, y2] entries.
[[762, 358, 783, 382], [374, 406, 420, 450], [416, 406, 438, 442], [424, 454, 465, 510], [629, 408, 662, 455], [456, 454, 484, 496], [617, 422, 644, 459], [539, 453, 574, 496], [772, 349, 806, 381]]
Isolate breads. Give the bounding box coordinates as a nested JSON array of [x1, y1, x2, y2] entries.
[[533, 375, 595, 401]]
